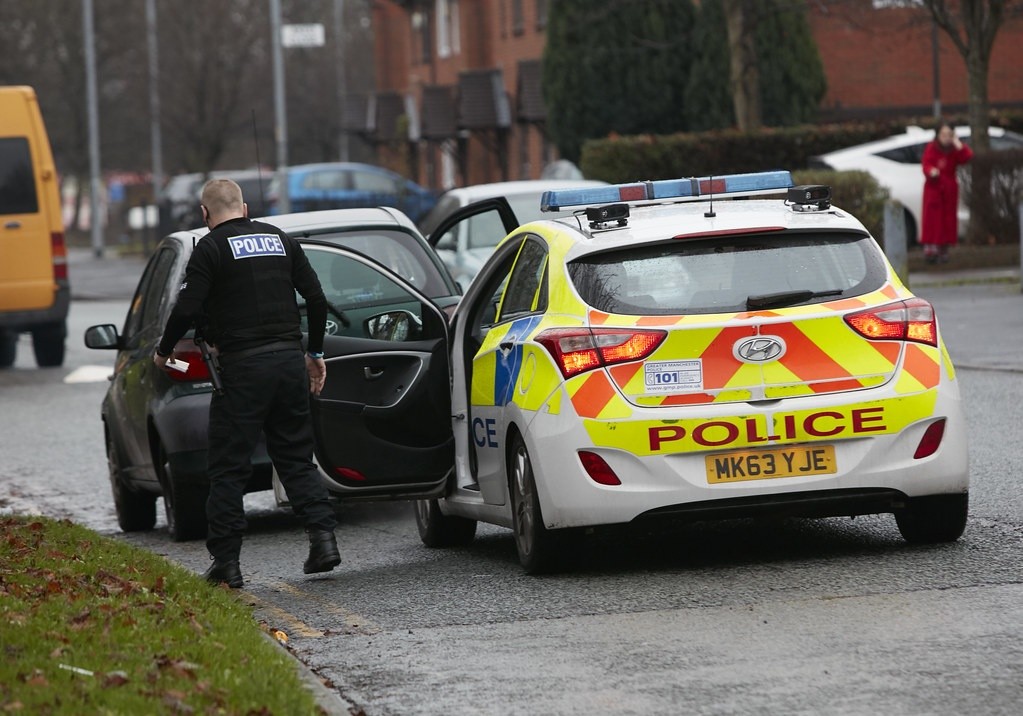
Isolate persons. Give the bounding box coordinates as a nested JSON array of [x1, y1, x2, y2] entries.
[[919, 121, 975, 270], [153, 179, 342, 589]]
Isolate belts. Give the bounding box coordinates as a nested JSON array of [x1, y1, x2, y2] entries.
[[215, 341, 303, 364]]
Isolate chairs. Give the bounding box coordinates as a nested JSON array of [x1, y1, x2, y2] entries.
[[324, 256, 384, 307]]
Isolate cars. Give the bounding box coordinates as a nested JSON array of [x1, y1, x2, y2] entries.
[[416, 178, 618, 298], [808, 125, 1023, 249], [59, 168, 280, 246], [261, 159, 444, 229], [268, 168, 968, 581], [84, 209, 464, 543]]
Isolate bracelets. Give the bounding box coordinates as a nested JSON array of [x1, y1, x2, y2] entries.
[[306, 350, 325, 358]]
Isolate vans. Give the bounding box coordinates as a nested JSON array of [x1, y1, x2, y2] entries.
[[0, 84, 72, 370]]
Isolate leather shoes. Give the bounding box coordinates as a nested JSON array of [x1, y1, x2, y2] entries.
[[201, 554, 245, 587], [303, 529, 341, 573]]
